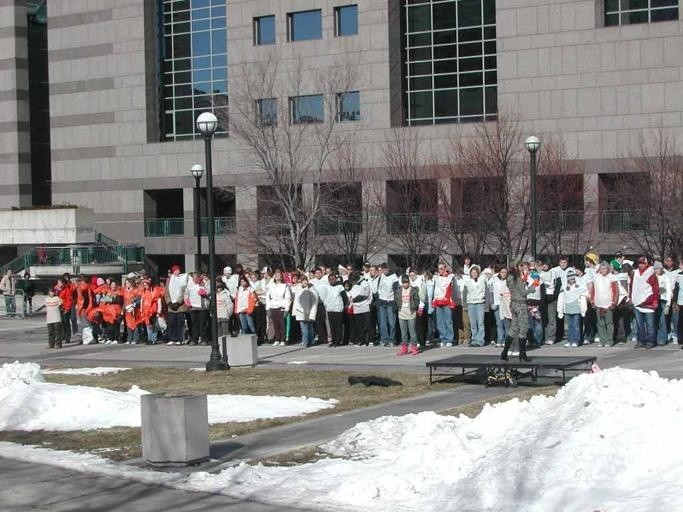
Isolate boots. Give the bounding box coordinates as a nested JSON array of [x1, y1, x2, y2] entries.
[[408, 341, 419, 354], [518, 337, 532, 363], [498, 335, 513, 363], [396, 343, 408, 355]]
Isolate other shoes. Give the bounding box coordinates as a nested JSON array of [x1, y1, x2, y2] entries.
[[545, 336, 679, 348], [272, 341, 286, 347], [425, 341, 505, 347], [166, 341, 211, 346], [86, 338, 162, 344]]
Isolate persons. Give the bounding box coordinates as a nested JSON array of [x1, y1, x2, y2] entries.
[[0, 268, 18, 318], [46, 252, 682, 362], [20, 271, 34, 317]]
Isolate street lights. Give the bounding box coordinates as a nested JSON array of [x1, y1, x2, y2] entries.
[[193, 109, 233, 373], [190, 162, 206, 276], [522, 134, 544, 260]]
